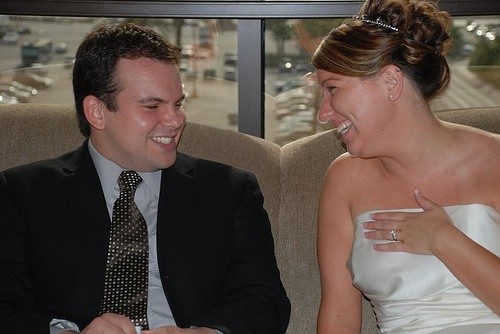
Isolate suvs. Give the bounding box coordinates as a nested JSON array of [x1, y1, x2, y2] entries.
[[278, 55, 293, 73], [223, 68, 237, 81]]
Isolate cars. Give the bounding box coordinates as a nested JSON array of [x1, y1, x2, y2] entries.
[[14, 73, 53, 89], [53, 43, 68, 54], [468, 21, 500, 41], [180, 43, 193, 59], [450, 26, 480, 56], [2, 33, 20, 45], [0, 91, 31, 103], [1, 99, 20, 106], [1, 81, 38, 95], [227, 111, 238, 122], [17, 64, 52, 75], [191, 28, 216, 59], [271, 78, 323, 147], [180, 63, 188, 72], [204, 69, 218, 82], [19, 26, 32, 34], [187, 68, 198, 79], [223, 51, 236, 65]]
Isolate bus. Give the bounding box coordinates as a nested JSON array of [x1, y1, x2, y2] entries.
[[20, 37, 54, 65]]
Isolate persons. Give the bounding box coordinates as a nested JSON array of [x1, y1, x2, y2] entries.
[[0, 21, 292, 334], [311, 0, 500, 334]]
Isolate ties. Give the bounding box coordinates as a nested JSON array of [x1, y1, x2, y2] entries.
[[99, 169, 149, 331]]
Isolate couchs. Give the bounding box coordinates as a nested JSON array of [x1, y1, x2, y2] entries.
[[1, 105, 500, 333]]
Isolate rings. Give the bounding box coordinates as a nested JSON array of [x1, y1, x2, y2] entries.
[[390, 230, 396, 240]]
